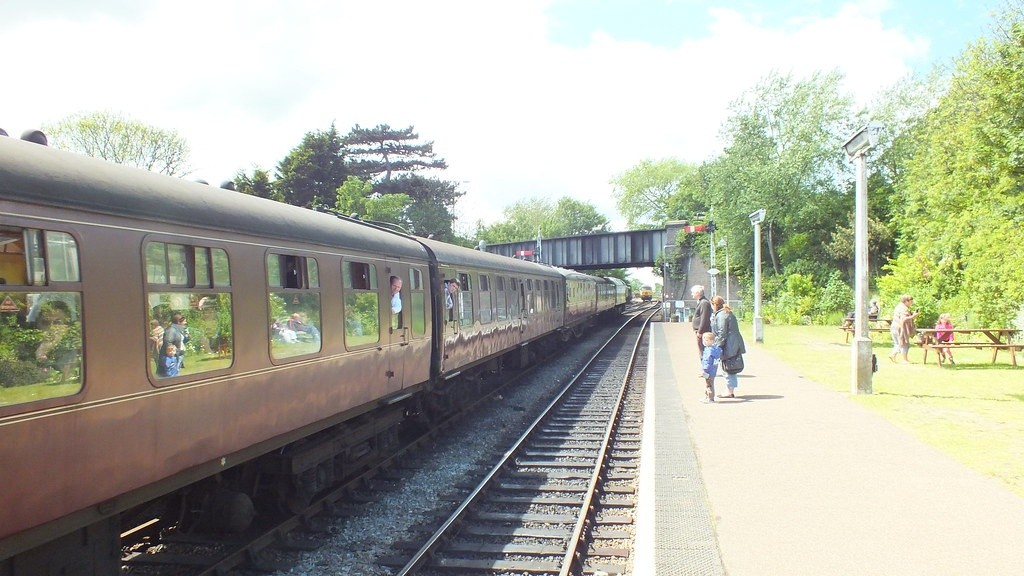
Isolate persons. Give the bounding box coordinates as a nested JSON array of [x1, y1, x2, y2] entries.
[[887, 293, 922, 365], [690, 284, 714, 379], [389, 275, 404, 317], [843, 311, 856, 336], [0, 298, 79, 400], [934, 313, 956, 365], [269, 303, 320, 351], [868, 298, 879, 321], [709, 295, 746, 401], [444, 277, 461, 311], [148, 292, 232, 375], [699, 331, 723, 406]]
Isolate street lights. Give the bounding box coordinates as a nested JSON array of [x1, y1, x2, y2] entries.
[[842, 125, 875, 394], [749, 209, 767, 341]]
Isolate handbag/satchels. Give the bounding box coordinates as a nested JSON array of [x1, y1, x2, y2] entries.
[[721, 347, 746, 374]]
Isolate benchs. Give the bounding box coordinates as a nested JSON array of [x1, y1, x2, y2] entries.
[[925, 343, 1024, 352], [840, 326, 883, 332], [845, 328, 891, 333], [916, 341, 1009, 349]]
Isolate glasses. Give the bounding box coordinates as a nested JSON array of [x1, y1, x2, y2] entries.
[[710, 297, 714, 305]]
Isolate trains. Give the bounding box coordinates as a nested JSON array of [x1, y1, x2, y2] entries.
[[640, 286, 653, 301], [0, 133, 626, 576]]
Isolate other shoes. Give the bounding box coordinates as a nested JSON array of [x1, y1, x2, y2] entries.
[[941, 355, 946, 363], [903, 360, 912, 364], [888, 354, 898, 364], [699, 397, 715, 404], [716, 393, 734, 398], [949, 356, 953, 363]]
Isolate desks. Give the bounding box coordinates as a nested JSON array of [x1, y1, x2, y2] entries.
[[841, 318, 894, 344], [917, 327, 1024, 367]]
